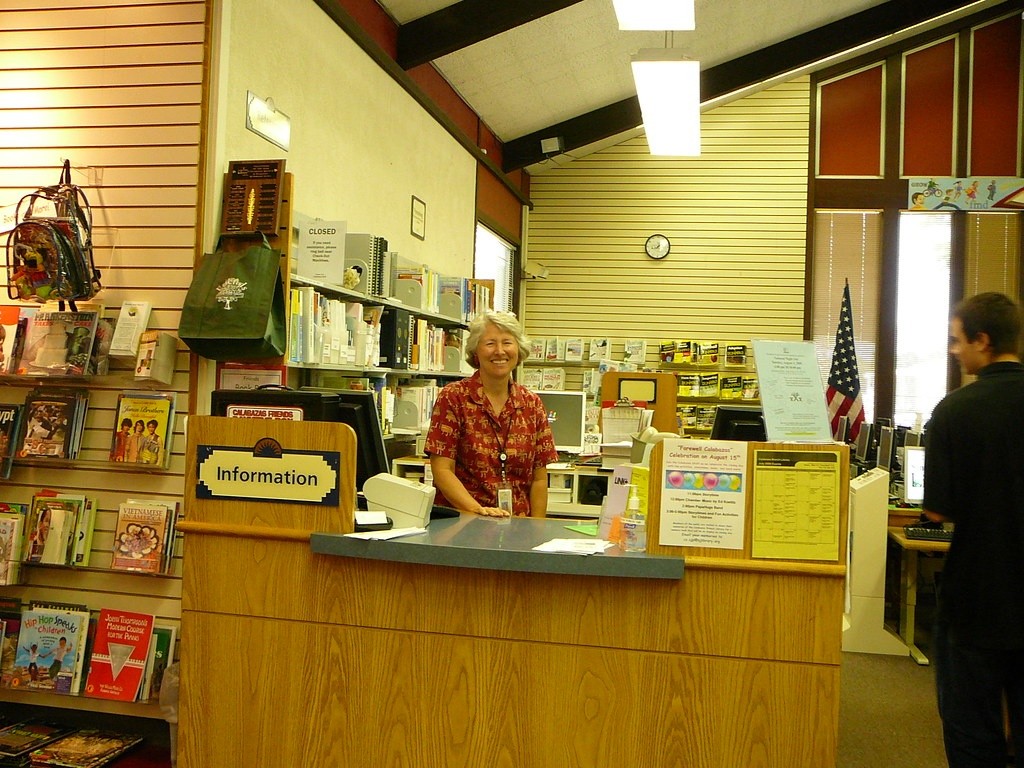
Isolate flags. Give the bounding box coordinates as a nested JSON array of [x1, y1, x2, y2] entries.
[[826, 283, 865, 444]]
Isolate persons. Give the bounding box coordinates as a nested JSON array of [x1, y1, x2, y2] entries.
[[22, 637, 73, 684], [65, 327, 98, 374], [113, 418, 164, 466], [922, 290, 1024, 768], [424, 308, 558, 518], [26, 508, 51, 562]]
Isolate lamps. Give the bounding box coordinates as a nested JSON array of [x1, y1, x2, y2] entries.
[[630, 31, 700, 159], [610, 0, 695, 32]]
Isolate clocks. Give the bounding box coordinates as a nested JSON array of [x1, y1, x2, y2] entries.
[[644, 234, 670, 261]]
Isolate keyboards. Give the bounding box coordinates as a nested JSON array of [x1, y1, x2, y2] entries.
[[903, 527, 954, 542]]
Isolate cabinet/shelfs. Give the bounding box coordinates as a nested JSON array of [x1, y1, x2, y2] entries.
[[523, 356, 608, 398], [660, 359, 761, 435], [212, 174, 496, 449]]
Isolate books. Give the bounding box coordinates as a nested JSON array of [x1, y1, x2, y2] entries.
[[300, 369, 444, 435], [522, 337, 748, 367], [0, 490, 181, 587], [109, 393, 171, 468], [0, 384, 89, 480], [0, 714, 147, 768], [519, 359, 759, 400], [288, 219, 489, 373], [0, 596, 178, 703], [0, 305, 102, 378], [676, 404, 718, 432]]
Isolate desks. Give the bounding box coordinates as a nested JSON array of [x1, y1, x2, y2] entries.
[[885, 506, 954, 666]]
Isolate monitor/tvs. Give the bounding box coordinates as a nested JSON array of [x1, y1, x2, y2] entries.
[[837, 417, 926, 504], [300, 385, 391, 492], [710, 406, 767, 442], [529, 390, 586, 453]]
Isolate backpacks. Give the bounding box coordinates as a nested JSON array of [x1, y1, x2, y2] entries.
[[6, 160, 102, 305]]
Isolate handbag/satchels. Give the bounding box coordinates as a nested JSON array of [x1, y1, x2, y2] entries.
[[178, 230, 286, 363]]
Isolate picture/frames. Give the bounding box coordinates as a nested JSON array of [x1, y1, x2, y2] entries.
[[410, 194, 426, 242]]
